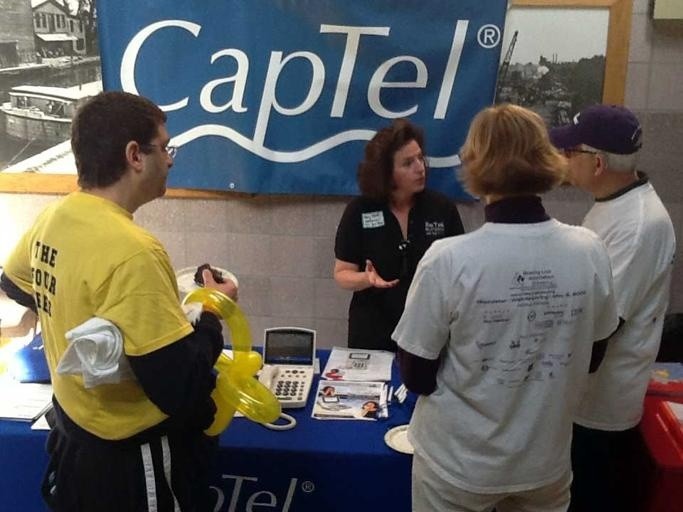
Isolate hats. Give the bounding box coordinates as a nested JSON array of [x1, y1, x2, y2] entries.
[[549, 105, 645, 152]]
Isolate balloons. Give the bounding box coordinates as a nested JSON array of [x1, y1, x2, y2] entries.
[[180, 285, 284, 439]]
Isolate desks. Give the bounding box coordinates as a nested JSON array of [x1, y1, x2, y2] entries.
[[0, 342, 406, 512]]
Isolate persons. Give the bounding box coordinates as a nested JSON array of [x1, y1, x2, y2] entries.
[[383, 98, 621, 511], [318, 385, 336, 397], [324, 368, 343, 379], [359, 400, 383, 420], [0, 86, 243, 512], [330, 116, 464, 355], [547, 95, 682, 510]]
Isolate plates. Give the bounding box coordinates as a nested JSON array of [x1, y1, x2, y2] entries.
[[175, 264, 241, 299]]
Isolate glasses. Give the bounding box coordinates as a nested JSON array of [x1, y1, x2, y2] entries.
[[138, 141, 179, 157]]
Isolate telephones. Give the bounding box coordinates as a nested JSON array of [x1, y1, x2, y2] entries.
[[258, 364, 315, 409]]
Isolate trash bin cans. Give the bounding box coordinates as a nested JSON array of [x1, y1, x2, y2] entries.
[[36, 55, 42, 64]]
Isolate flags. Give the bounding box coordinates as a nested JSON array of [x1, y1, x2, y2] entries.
[[94, 0, 508, 205]]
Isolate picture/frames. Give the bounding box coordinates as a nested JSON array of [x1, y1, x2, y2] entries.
[[0, 2, 635, 198]]
